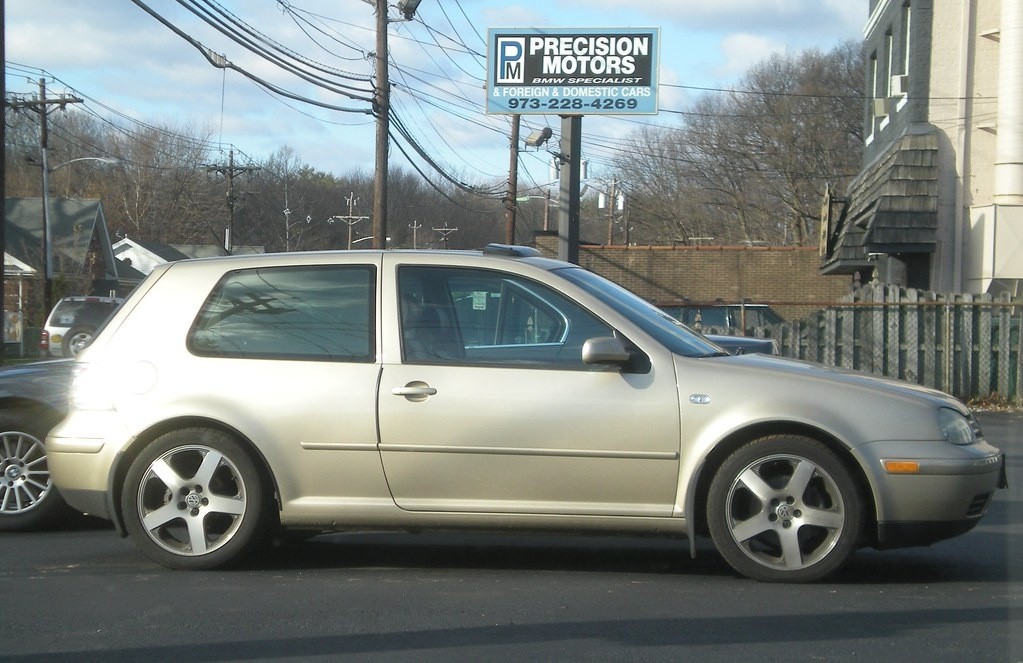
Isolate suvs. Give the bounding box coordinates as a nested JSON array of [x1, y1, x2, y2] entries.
[[40, 290, 128, 363], [45, 243, 1009, 586]]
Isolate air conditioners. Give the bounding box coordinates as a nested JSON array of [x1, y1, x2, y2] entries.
[[889, 76, 908, 97], [872, 98, 889, 119]]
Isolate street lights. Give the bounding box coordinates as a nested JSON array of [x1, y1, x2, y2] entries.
[[41, 156, 124, 315]]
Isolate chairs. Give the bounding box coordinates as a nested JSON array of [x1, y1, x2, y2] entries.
[[400, 291, 460, 362]]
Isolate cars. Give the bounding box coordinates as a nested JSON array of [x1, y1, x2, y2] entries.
[[446, 274, 780, 370], [0, 347, 97, 535]]
